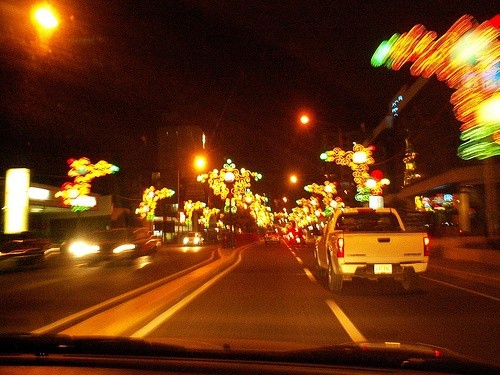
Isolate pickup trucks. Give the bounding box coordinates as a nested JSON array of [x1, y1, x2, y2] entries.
[[313, 207, 430, 295]]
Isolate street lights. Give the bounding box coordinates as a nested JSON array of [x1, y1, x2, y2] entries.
[[224, 171, 235, 247], [300, 114, 344, 186], [177, 154, 206, 245]]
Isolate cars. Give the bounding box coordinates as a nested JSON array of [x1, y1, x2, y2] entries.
[[263, 231, 280, 245], [180, 231, 205, 247], [81, 226, 162, 263]]
